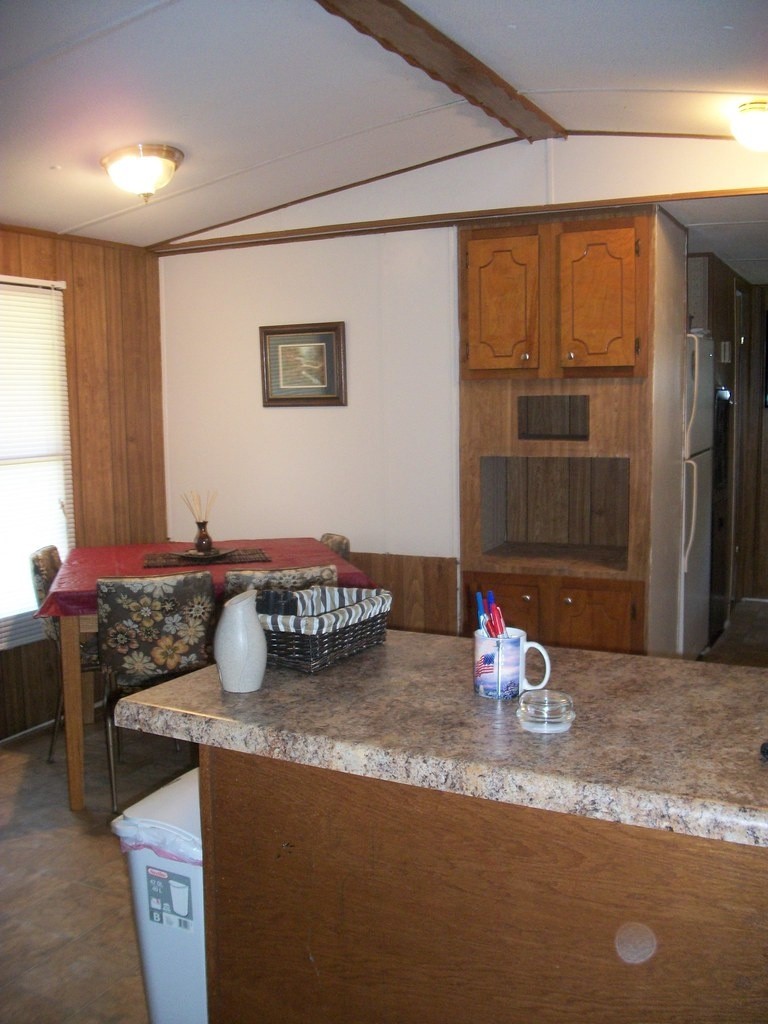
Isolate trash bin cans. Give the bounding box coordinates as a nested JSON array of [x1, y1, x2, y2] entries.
[[109, 765, 208, 1024]]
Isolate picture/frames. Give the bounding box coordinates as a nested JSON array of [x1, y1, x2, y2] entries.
[[259, 321, 348, 406]]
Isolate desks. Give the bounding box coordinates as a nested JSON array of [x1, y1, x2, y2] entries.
[[113, 629, 768, 1024]]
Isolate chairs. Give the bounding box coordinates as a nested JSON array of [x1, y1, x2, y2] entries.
[[30, 544, 102, 763], [222, 564, 340, 605], [95, 569, 217, 812]]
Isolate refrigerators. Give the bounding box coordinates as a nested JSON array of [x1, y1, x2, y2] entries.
[[677, 334, 714, 661]]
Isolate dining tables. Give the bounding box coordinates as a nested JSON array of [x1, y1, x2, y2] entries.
[[51, 538, 370, 810]]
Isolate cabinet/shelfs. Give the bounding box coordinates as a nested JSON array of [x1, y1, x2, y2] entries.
[[456, 204, 656, 380], [461, 570, 645, 657]]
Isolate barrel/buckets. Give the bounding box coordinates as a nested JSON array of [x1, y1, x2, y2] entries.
[[109, 767, 210, 1024]]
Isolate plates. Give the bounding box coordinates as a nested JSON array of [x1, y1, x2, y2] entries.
[[168, 549, 239, 559]]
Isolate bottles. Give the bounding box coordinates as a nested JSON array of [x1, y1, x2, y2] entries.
[[214, 590, 268, 692]]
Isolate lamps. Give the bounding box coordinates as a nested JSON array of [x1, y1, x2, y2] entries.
[[100, 144, 186, 204]]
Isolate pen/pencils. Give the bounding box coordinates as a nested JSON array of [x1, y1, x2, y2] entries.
[[476, 590, 509, 639]]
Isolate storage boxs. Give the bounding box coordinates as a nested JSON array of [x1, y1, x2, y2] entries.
[[257, 584, 392, 675]]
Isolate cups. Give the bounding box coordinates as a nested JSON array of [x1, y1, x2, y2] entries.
[[474, 626, 551, 701]]
